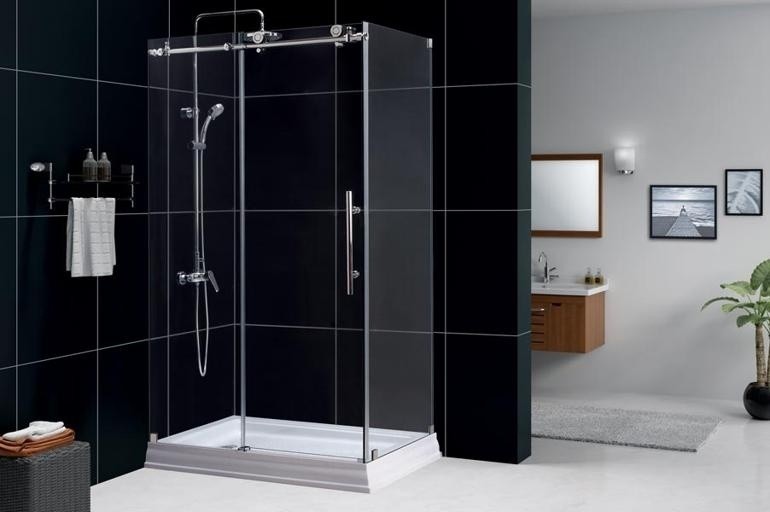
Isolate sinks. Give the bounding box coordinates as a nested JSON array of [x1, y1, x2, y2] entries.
[[532, 282, 581, 290]]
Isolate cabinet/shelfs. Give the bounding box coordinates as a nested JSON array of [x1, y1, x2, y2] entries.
[[530, 291, 611, 355]]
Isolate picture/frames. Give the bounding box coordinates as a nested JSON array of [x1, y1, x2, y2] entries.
[[723, 167, 765, 219], [647, 183, 719, 241]]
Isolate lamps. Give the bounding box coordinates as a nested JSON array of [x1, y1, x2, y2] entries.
[[613, 143, 637, 176]]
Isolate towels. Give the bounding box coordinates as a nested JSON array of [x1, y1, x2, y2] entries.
[[64, 194, 119, 280], [2, 419, 67, 443], [0, 425, 78, 458]]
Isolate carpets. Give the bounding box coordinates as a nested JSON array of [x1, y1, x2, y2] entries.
[[531, 400, 727, 454]]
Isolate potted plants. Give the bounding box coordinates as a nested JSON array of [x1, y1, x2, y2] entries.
[[698, 257, 770, 421]]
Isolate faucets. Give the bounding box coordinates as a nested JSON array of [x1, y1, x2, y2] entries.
[[537, 251, 550, 283]]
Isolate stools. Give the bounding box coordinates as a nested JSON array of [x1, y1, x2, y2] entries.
[[0, 440, 94, 511]]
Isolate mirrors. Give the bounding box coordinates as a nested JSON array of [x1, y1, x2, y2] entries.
[[531, 151, 604, 238]]
